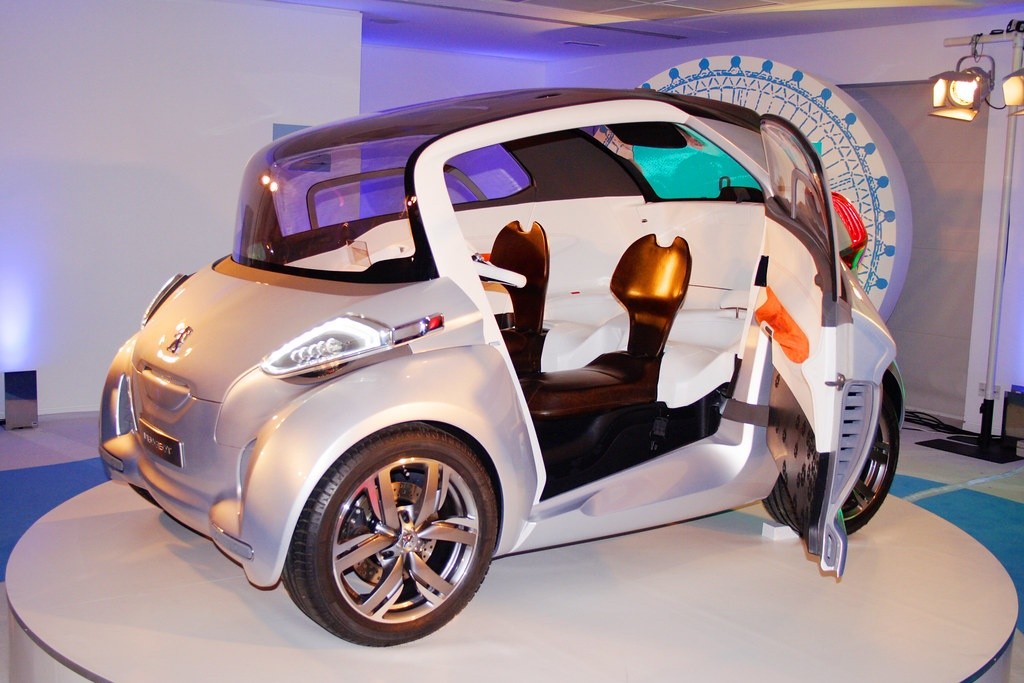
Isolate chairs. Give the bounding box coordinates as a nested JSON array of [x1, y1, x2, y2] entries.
[[489, 220, 551, 379], [519, 233, 693, 425]]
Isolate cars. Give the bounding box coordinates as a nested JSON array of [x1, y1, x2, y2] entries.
[[100, 86, 906, 645]]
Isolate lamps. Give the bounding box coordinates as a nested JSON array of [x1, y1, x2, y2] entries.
[[1001, 69, 1024, 118], [928, 55, 995, 122]]
